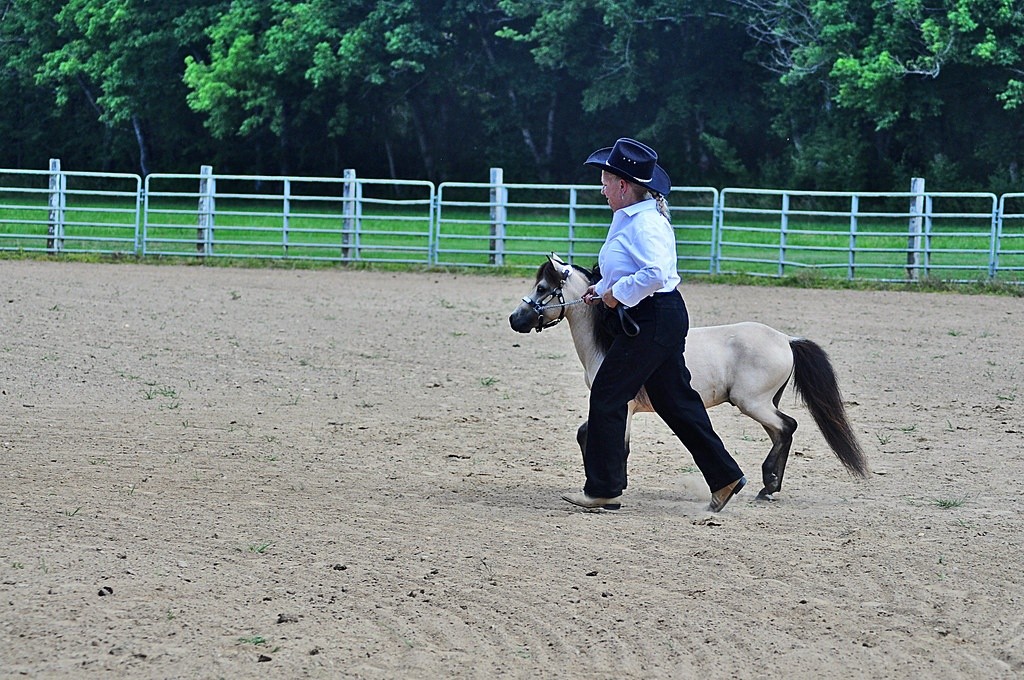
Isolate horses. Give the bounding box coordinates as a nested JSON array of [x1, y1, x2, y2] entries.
[[508, 250, 871, 503]]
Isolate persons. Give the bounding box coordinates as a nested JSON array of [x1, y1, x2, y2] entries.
[[562, 138, 747, 513]]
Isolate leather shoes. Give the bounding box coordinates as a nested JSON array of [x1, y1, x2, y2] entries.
[[562, 491, 623, 510], [703, 476, 747, 513]]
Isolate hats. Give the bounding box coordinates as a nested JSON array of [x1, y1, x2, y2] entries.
[[582, 138, 671, 196]]
